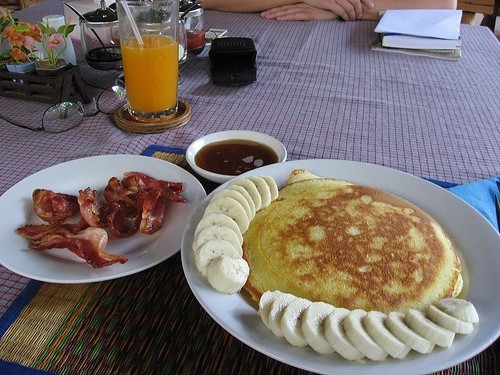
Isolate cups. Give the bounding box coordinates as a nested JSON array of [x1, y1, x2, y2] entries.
[[116, 0, 179, 123], [79, 0, 123, 69], [179, 20, 187, 64], [179, 1, 206, 54]]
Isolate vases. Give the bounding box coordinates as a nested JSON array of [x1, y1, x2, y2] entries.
[[35, 58, 66, 76], [6, 56, 37, 73]]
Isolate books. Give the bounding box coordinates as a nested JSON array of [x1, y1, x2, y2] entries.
[[371, 8, 463, 62]]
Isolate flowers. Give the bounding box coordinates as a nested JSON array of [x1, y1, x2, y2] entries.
[[0, 8, 42, 63], [36, 16, 77, 66]]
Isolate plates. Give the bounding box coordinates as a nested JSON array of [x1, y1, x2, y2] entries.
[[181, 159, 500, 375], [186, 130, 287, 183], [0, 155, 207, 283]]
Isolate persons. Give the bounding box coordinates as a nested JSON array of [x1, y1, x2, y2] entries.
[[194, 0, 457, 21]]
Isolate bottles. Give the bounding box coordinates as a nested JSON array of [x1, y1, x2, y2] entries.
[[40, 15, 76, 66]]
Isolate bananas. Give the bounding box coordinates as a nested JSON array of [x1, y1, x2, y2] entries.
[[259, 290, 479, 361], [192, 176, 279, 294]]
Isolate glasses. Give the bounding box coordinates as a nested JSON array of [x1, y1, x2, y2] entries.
[[0, 69, 128, 133]]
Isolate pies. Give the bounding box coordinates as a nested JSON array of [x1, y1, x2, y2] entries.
[[240, 170, 463, 312]]
